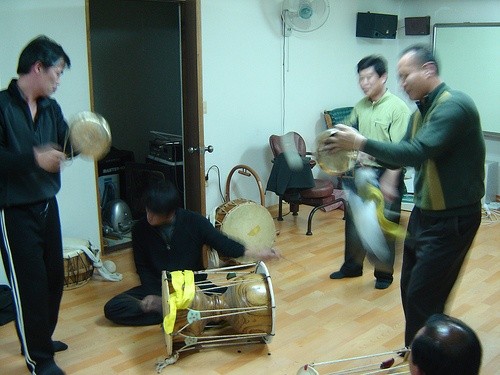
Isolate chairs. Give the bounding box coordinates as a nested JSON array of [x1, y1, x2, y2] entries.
[[270, 131, 347, 236], [323, 106, 358, 192]]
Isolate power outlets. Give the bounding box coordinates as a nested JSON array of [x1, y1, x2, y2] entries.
[[205, 172, 208, 187]]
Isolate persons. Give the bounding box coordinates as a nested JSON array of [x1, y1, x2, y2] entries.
[[328, 55, 411, 289], [103, 181, 283, 327], [0, 32, 109, 375], [317, 45, 486, 359], [407, 314, 483, 375]]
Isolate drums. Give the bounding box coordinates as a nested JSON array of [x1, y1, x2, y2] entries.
[[162, 261, 276, 355], [214, 198, 276, 264], [315, 129, 357, 175], [61, 237, 95, 289], [69, 111, 112, 161]]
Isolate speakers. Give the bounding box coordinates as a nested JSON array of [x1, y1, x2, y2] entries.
[[404, 16, 431, 35], [355, 12, 398, 39]]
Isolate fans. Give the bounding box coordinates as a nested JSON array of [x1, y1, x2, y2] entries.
[[280, 0, 330, 37]]
